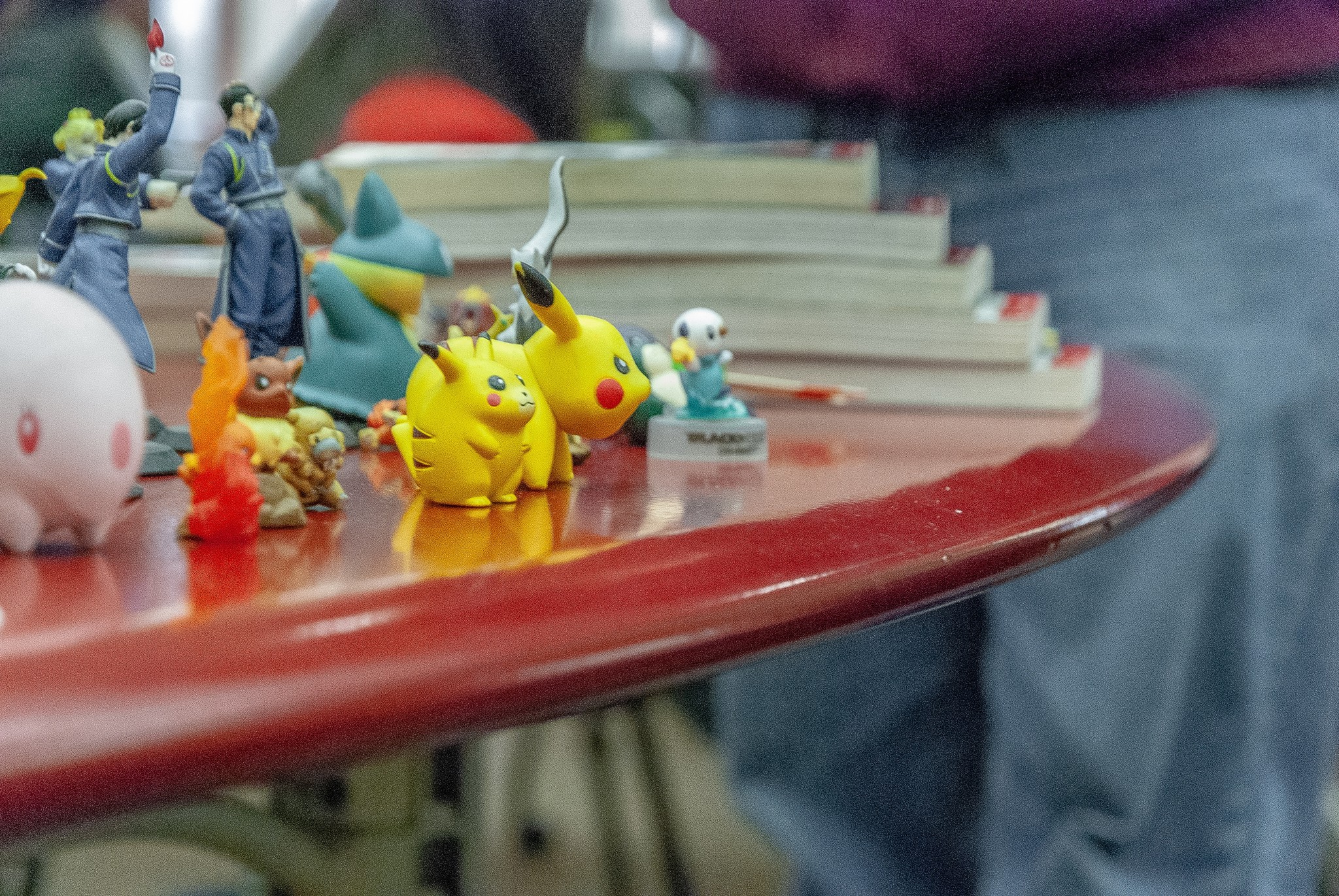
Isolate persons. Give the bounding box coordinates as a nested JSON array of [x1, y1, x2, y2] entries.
[[0, 0, 794, 896], [672, 307, 735, 407], [671, 0, 1339, 896], [190, 78, 307, 388], [38, 44, 181, 500]]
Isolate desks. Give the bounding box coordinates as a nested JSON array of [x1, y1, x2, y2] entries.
[[1, 360, 1223, 896]]
